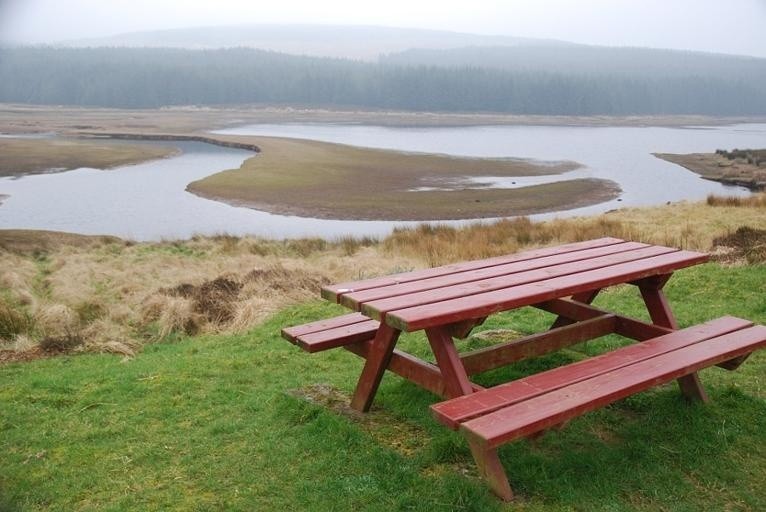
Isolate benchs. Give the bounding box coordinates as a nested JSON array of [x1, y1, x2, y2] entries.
[[281, 311, 381, 353], [428, 316, 766, 449]]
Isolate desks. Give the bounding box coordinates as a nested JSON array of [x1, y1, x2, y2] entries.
[[321, 237, 708, 501]]
